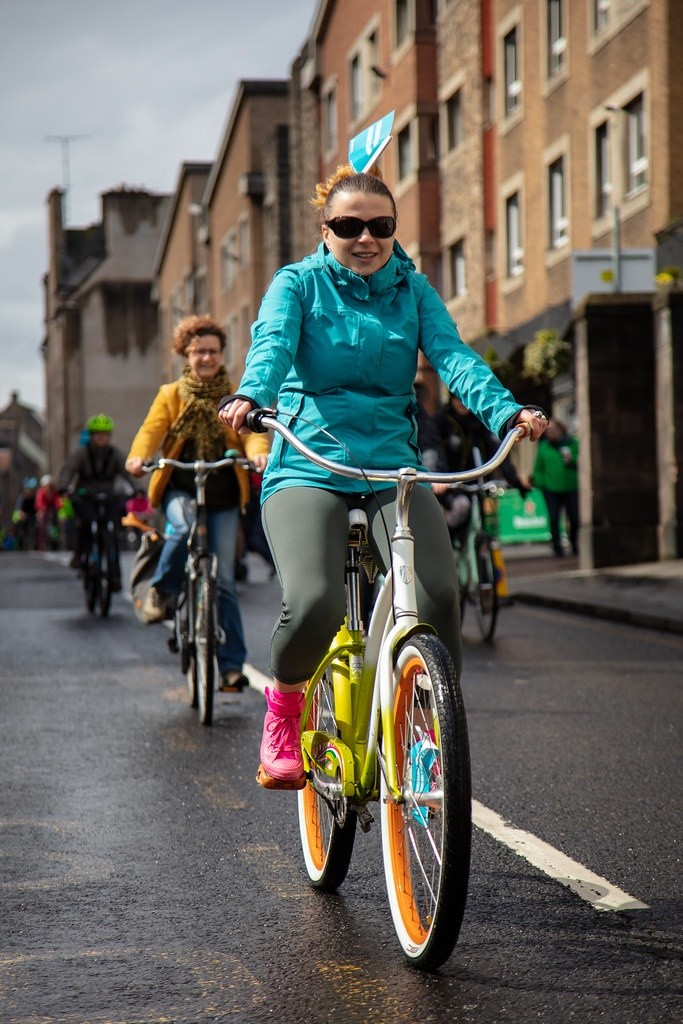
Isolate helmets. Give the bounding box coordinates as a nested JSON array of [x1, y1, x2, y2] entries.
[[86, 414, 115, 432]]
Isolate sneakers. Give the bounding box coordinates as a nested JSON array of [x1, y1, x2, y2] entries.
[[259, 685, 307, 781]]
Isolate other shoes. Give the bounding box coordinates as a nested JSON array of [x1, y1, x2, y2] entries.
[[219, 674, 249, 688], [142, 587, 167, 622]]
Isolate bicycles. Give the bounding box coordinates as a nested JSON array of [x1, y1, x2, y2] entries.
[[432, 482, 518, 641], [61, 483, 134, 618], [242, 409, 549, 969], [140, 448, 254, 728]]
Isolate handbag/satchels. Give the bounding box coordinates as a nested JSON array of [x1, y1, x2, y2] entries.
[[122, 513, 173, 625]]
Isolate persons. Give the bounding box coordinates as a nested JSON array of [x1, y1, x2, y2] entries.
[[12, 473, 153, 552], [410, 379, 443, 471], [124, 314, 271, 689], [57, 413, 137, 594], [215, 169, 547, 818], [532, 419, 580, 558], [419, 388, 530, 547]]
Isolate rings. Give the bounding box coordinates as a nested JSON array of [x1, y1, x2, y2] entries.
[[534, 410, 543, 419], [541, 415, 546, 420], [223, 404, 231, 411]]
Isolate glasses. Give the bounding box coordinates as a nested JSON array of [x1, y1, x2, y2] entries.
[[322, 215, 397, 239]]
